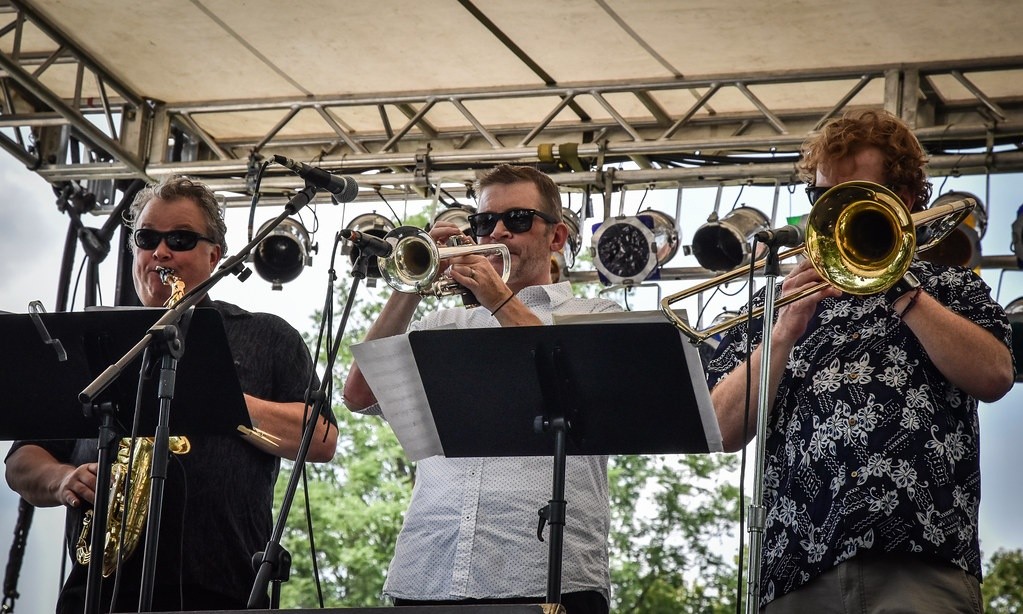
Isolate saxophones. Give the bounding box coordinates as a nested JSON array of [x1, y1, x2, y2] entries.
[[76, 268, 194, 576]]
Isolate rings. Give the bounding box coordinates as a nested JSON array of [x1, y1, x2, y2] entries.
[[469, 269, 475, 278]]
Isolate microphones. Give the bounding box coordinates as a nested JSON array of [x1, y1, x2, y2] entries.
[[754, 214, 810, 247], [340, 229, 392, 259], [273, 155, 359, 202]]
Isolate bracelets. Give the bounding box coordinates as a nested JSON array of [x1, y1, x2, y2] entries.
[[896, 287, 923, 320], [491, 292, 514, 316]]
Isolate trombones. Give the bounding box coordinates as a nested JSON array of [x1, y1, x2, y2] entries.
[[658, 180, 977, 339]]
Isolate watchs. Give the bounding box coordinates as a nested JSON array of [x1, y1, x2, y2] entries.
[[885, 271, 920, 309]]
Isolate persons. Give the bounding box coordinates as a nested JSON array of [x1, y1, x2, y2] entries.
[[3, 175, 338, 614], [705, 109, 1023, 614], [345, 162, 624, 614]]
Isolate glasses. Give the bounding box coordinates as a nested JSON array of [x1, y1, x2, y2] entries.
[[134, 228, 216, 252], [805, 184, 832, 206], [468, 208, 558, 237]]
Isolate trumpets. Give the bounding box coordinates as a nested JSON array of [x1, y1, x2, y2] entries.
[[377, 228, 514, 298]]
